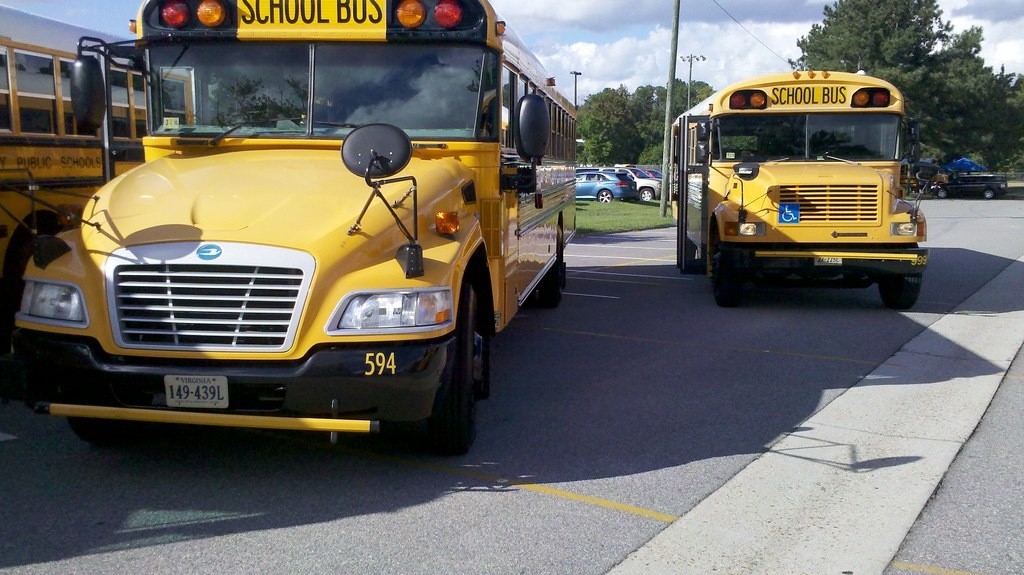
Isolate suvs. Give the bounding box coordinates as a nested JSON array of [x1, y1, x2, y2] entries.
[[930, 174, 1008, 199], [575, 172, 638, 204]]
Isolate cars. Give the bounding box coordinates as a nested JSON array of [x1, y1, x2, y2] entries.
[[575, 164, 669, 203]]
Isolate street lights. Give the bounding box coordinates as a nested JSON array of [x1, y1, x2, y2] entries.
[[680, 54, 706, 109], [570, 71, 581, 107]]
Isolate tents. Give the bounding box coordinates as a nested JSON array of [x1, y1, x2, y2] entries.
[[938, 157, 989, 173]]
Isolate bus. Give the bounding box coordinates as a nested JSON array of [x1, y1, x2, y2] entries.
[[0, 5, 195, 394], [670, 70, 940, 309], [0, 0, 578, 459]]
